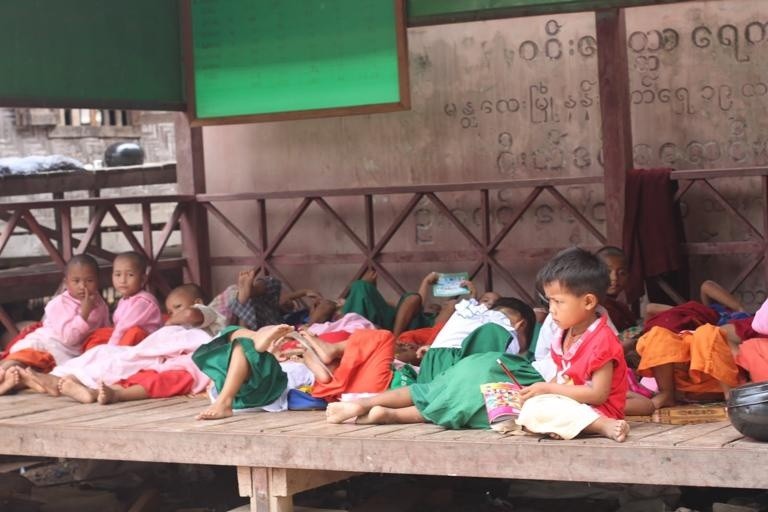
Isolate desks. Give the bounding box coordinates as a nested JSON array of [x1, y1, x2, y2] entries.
[[0, 169, 97, 264], [97, 160, 183, 266]]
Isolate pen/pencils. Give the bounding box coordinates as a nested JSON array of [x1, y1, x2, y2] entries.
[[496, 359, 522, 389]]
